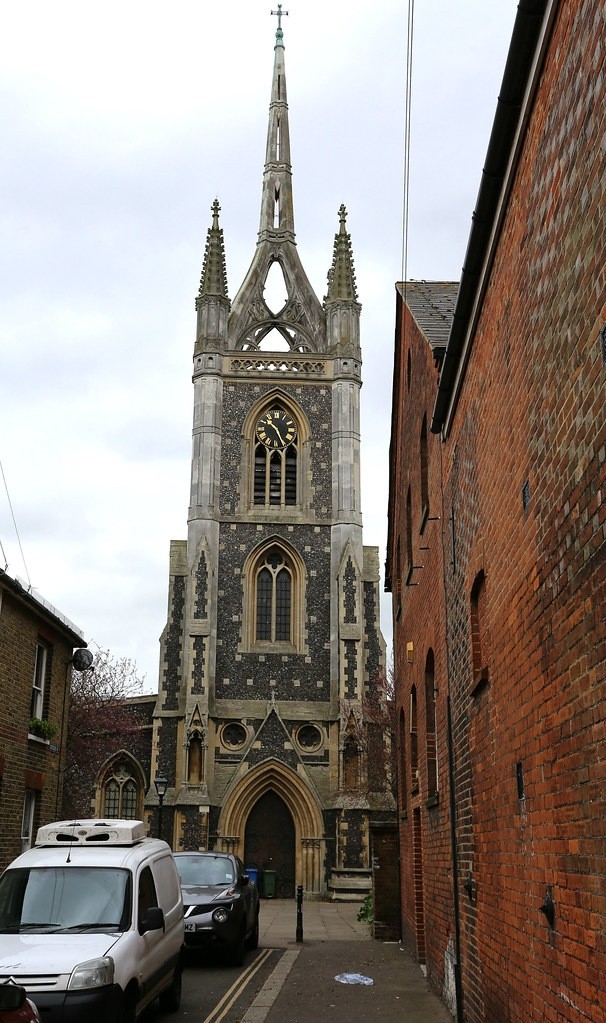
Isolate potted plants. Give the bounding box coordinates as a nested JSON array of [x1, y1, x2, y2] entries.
[[29, 717, 58, 742]]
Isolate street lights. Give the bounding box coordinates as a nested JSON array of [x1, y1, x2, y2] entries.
[[153, 768, 170, 839]]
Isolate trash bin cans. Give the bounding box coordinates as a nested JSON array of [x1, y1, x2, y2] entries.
[[264, 870, 276, 898], [246, 869, 258, 885]]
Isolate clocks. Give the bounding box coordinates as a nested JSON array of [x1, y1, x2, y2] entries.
[[255, 410, 298, 449]]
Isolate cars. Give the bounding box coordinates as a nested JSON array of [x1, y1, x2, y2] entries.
[[171, 850, 260, 969]]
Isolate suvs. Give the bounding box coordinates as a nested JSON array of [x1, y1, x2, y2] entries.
[[0, 818, 186, 1023]]
